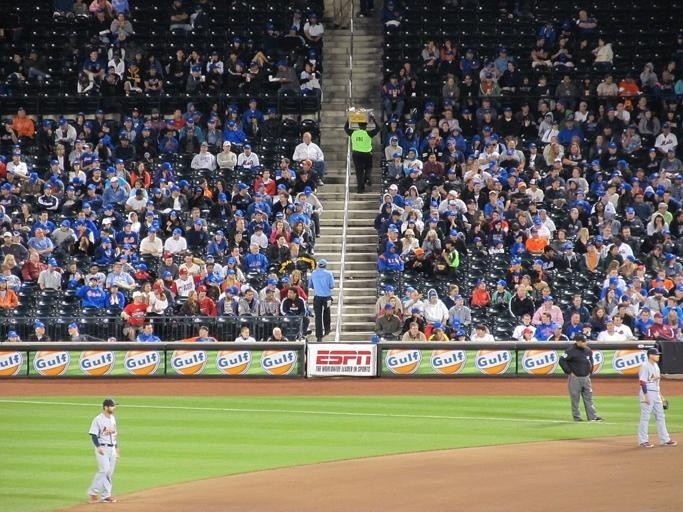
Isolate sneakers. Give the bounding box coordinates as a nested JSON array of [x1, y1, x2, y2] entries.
[[316, 177, 324, 186], [101, 496, 116, 502], [88, 495, 98, 503], [575, 418, 585, 422], [640, 442, 654, 448], [588, 416, 603, 422], [662, 440, 678, 446]]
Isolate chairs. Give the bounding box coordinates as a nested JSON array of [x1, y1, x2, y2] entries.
[[383, 2, 682, 339], [0, 0, 322, 343]]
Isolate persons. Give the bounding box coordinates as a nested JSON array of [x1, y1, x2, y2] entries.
[[638, 348, 677, 449], [361, 0, 683, 343], [87, 399, 119, 503], [559, 335, 603, 421], [343, 114, 380, 193], [1, 0, 334, 343]]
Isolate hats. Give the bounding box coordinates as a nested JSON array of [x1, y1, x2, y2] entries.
[[576, 335, 588, 342], [34, 322, 45, 328], [647, 348, 663, 355], [103, 399, 119, 406], [8, 331, 18, 337], [383, 48, 682, 333], [318, 259, 327, 267], [0, 97, 312, 300], [67, 324, 78, 328]]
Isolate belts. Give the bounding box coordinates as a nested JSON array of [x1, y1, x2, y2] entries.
[[101, 444, 114, 447]]
